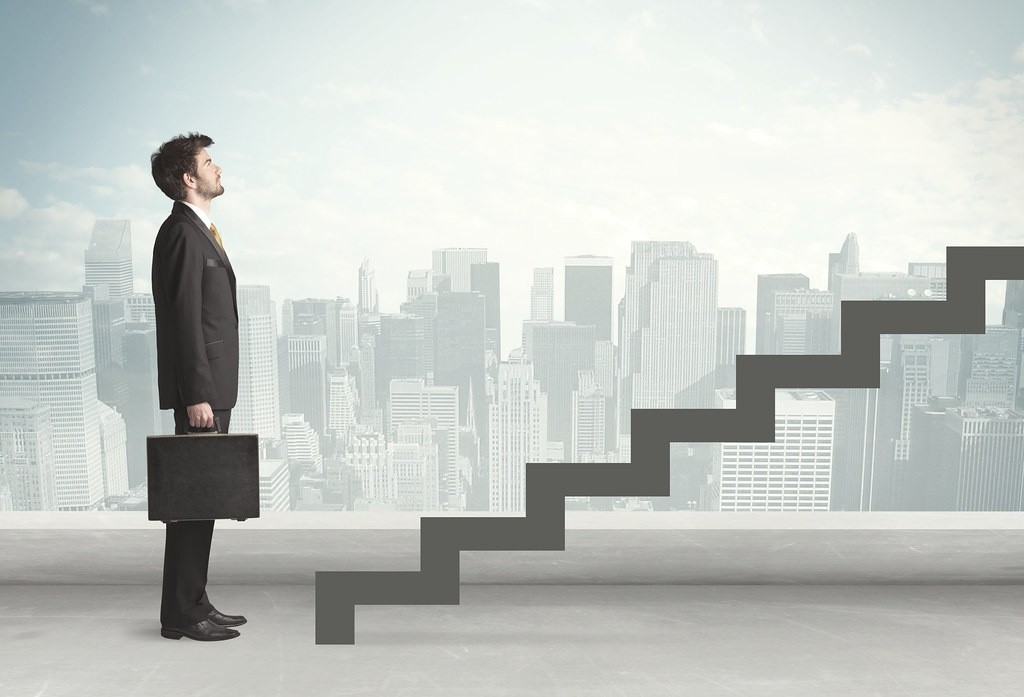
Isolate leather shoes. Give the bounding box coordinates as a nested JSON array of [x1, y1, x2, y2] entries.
[[160, 617, 240, 642], [208, 607, 247, 628]]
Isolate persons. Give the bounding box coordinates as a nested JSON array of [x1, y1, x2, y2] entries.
[[152, 134, 247, 640]]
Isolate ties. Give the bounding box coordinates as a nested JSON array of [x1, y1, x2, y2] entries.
[[210, 222, 225, 255]]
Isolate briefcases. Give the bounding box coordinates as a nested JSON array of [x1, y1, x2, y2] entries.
[[146, 416, 262, 523]]
